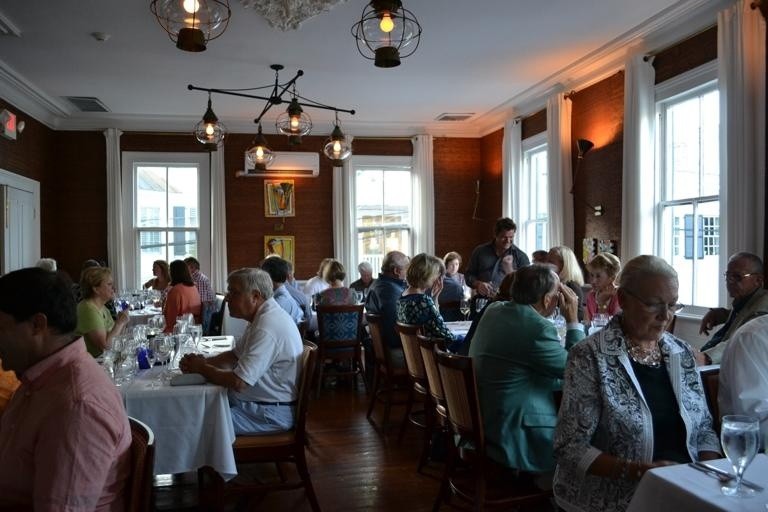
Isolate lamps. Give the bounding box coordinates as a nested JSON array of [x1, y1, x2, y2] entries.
[[569, 138, 605, 217], [350, 0, 422, 66], [187, 65, 355, 170], [150, 1, 232, 53]]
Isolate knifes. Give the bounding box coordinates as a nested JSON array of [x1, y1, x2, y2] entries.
[[695, 461, 764, 493]]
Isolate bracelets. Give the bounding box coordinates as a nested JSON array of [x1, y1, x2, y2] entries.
[[624, 457, 632, 480], [636, 459, 641, 481]]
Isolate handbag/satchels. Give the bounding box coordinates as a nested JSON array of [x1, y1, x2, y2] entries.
[[200, 299, 223, 336]]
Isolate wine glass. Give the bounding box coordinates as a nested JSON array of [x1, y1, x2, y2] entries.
[[354, 291, 363, 305], [460, 299, 470, 329], [114, 289, 162, 314], [364, 288, 369, 298], [593, 313, 609, 332], [476, 298, 488, 314], [103, 312, 203, 390], [720, 414, 761, 499]]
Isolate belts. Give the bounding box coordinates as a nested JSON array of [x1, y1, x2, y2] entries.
[[256, 401, 292, 405]]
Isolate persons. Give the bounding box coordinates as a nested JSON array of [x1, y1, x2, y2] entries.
[[35, 257, 57, 272], [551, 254, 724, 512], [143, 257, 216, 333], [365, 217, 623, 511], [717, 313, 768, 454], [181, 268, 305, 511], [0, 268, 133, 512], [694, 252, 768, 366], [74, 256, 130, 357], [263, 255, 376, 386]]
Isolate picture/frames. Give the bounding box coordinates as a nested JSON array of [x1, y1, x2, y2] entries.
[[264, 179, 296, 219], [264, 235, 296, 267]]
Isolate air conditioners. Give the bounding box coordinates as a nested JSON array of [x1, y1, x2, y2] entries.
[[244, 151, 320, 178]]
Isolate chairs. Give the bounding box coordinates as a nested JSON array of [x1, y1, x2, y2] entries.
[[316, 304, 365, 401], [700, 368, 719, 436], [416, 334, 447, 473], [433, 344, 555, 511], [395, 321, 426, 450], [129, 416, 156, 512], [365, 313, 425, 428], [214, 345, 322, 512]]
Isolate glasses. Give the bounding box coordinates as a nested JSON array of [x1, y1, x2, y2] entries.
[[722, 272, 752, 281], [628, 295, 685, 314]]
